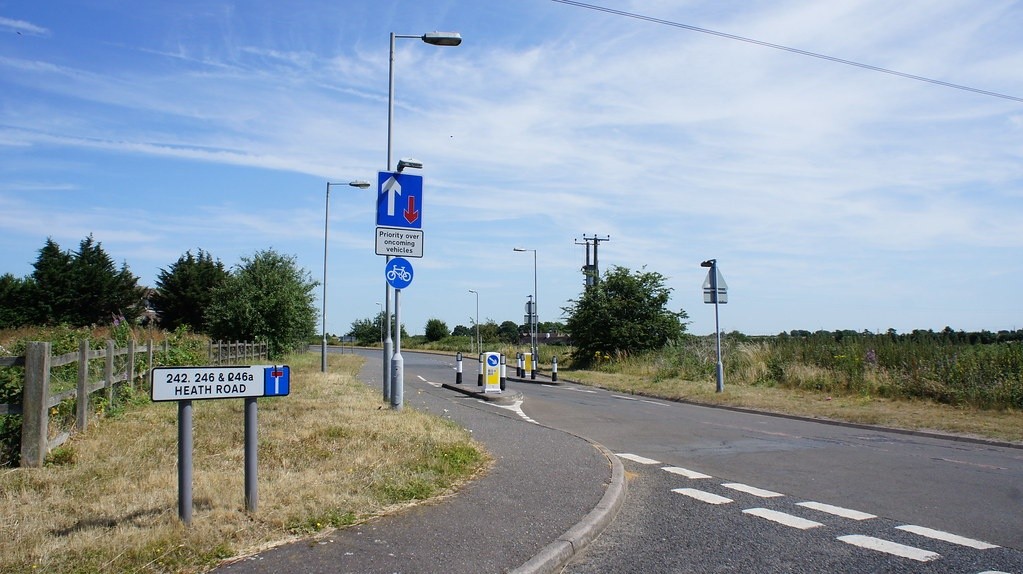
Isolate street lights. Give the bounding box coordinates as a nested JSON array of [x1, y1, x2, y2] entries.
[[470, 248, 725, 394], [317, 30, 461, 411]]
